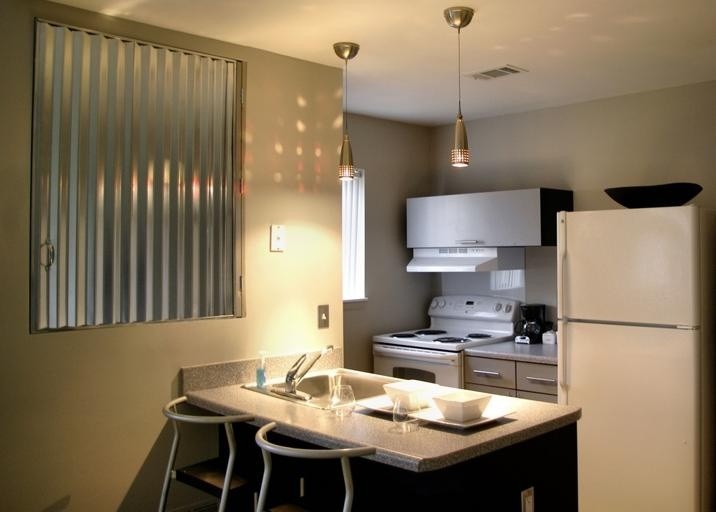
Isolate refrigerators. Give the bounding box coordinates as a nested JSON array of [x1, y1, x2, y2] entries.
[[556, 204, 716, 512]]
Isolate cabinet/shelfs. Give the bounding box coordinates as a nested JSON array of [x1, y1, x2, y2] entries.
[[406, 187, 574, 249], [463, 355, 517, 402], [516, 361, 558, 404]]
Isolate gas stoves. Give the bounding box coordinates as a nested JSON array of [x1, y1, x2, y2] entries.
[[371, 328, 511, 352]]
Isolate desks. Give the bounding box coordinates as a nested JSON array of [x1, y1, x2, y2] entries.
[[184, 367, 582, 512]]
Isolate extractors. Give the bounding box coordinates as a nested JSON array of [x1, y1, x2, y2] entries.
[[406, 248, 527, 273]]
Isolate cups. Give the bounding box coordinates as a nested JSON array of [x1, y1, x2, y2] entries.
[[329, 384, 357, 418], [392, 396, 422, 431]]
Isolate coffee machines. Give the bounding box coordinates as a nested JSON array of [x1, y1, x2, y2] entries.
[[514, 304, 553, 345]]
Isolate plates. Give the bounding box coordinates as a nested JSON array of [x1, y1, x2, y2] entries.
[[354, 388, 519, 428]]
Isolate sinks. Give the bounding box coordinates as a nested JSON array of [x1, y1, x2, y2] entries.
[[242, 368, 401, 410]]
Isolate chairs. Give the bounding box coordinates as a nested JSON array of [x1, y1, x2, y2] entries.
[[157, 394, 255, 512], [245, 421, 376, 511]]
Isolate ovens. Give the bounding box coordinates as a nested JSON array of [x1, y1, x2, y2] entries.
[[372, 343, 462, 389]]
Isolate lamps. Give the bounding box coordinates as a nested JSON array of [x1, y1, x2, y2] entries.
[[444, 5, 475, 168], [333, 42, 360, 180]]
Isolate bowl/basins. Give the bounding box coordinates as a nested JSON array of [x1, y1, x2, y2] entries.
[[382, 379, 435, 410], [604, 182, 705, 209], [432, 390, 493, 423]]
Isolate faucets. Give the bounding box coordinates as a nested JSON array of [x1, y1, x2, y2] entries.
[[285, 345, 335, 393]]
